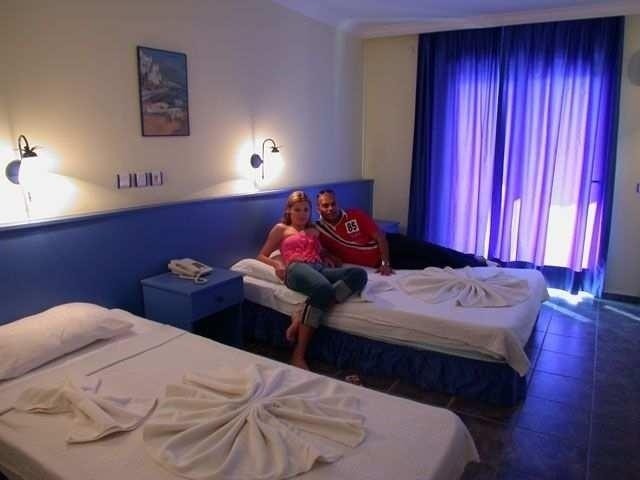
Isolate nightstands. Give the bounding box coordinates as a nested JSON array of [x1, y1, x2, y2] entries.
[[375, 218, 400, 234], [141, 266, 243, 348]]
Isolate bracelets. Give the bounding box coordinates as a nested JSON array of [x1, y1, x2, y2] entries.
[[380, 257, 390, 266]]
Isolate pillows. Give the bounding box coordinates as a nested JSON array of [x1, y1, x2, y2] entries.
[[231, 251, 284, 284], [0, 301, 134, 380]]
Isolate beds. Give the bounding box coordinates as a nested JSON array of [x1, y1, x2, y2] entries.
[[0, 307, 481, 480], [243, 263, 551, 407]]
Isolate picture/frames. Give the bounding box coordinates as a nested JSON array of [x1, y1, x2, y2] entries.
[[137, 45, 190, 137]]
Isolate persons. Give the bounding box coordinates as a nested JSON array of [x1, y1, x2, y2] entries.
[[307, 188, 487, 277], [257, 189, 370, 373]]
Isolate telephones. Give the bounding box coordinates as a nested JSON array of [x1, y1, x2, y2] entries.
[[167, 257, 213, 276]]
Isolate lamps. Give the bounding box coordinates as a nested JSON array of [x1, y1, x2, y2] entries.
[[6, 134, 38, 185], [250, 139, 280, 180]]
